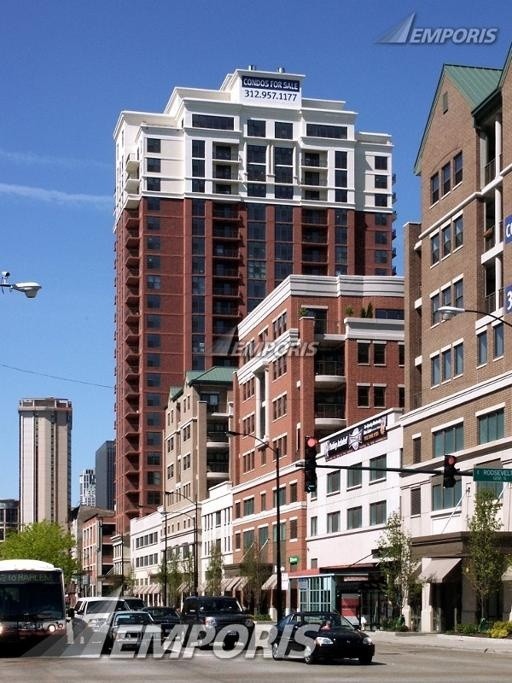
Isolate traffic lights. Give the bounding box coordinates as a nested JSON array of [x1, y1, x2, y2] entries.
[[304, 435, 318, 492], [442, 454, 457, 488]]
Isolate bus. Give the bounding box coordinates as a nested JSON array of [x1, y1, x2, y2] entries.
[[0, 558, 74, 647]]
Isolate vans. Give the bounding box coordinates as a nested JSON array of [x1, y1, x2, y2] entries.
[[177, 594, 256, 650]]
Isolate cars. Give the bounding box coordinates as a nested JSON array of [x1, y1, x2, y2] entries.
[[71, 596, 181, 653], [269, 610, 376, 665]]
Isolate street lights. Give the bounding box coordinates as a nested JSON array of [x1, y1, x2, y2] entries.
[[164, 490, 199, 596], [223, 430, 282, 623]]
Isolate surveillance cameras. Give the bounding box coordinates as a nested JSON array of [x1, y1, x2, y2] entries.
[[1, 271, 10, 279]]
